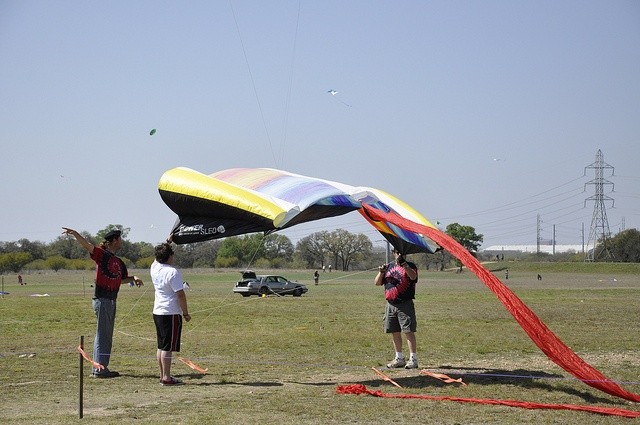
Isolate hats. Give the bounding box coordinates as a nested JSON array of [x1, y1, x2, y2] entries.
[[104, 230, 121, 239]]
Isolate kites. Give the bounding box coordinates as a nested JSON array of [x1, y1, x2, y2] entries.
[[149, 128, 157, 135], [328, 89, 354, 110], [157, 166, 640, 419]]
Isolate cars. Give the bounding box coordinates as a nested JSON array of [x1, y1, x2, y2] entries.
[[233, 271, 307, 297]]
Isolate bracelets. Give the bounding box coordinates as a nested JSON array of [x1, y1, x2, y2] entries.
[[166, 238, 172, 244], [183, 313, 189, 316], [403, 265, 411, 269]]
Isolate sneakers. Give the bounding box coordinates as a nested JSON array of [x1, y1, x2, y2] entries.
[[91, 367, 119, 378], [405, 357, 418, 369], [387, 357, 406, 367], [159, 376, 184, 385]]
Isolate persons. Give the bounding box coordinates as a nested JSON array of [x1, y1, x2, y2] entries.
[[328, 263, 332, 272], [129, 280, 134, 288], [149, 232, 192, 385], [537, 274, 542, 281], [373, 245, 418, 369], [505, 270, 509, 280], [501, 252, 505, 261], [496, 254, 499, 261], [322, 265, 326, 272], [17, 274, 23, 285], [312, 270, 319, 280], [458, 265, 461, 274], [61, 226, 145, 379]]
